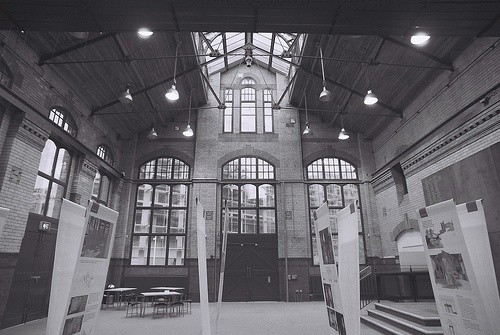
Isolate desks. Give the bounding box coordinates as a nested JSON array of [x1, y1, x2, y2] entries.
[[140, 292, 180, 318], [151, 287, 185, 306], [104, 288, 137, 309]]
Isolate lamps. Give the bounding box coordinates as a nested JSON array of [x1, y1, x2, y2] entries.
[[183, 95, 194, 137], [165, 44, 179, 101], [119, 60, 132, 102], [364, 62, 378, 105], [319, 47, 333, 101], [149, 127, 157, 136], [302, 93, 313, 136], [338, 113, 349, 139]]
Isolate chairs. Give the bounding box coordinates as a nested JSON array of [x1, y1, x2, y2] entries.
[[101, 289, 191, 319]]
